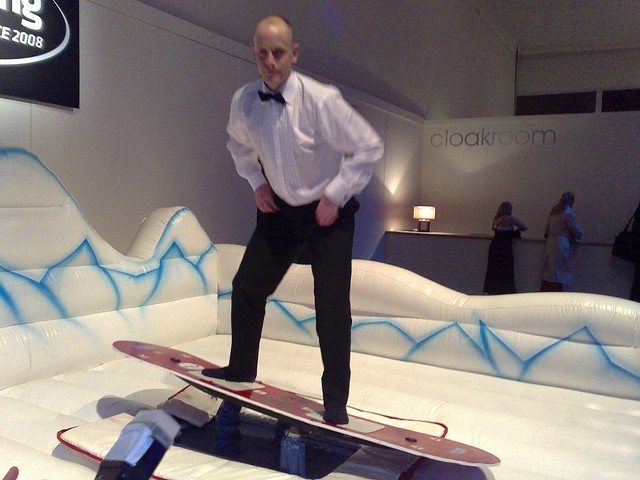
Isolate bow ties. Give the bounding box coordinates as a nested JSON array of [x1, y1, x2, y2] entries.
[[258, 90, 285, 105]]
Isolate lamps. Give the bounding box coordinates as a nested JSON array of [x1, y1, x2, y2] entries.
[[414, 205, 435, 232]]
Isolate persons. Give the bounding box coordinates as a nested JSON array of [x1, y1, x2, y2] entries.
[[629, 204, 640, 304], [484, 201, 528, 295], [540, 188, 583, 292], [200, 14, 387, 428]]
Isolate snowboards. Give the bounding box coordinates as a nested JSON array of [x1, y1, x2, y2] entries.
[[114, 340, 502, 467]]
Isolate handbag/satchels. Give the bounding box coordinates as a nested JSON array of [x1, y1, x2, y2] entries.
[[611, 205, 640, 263]]
